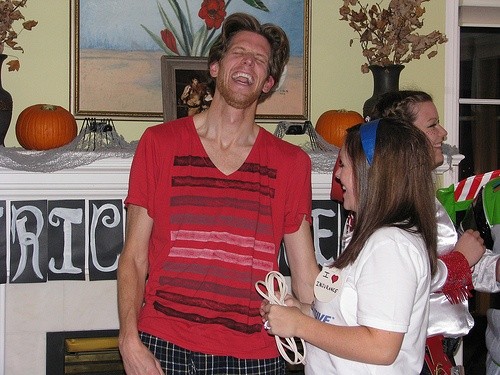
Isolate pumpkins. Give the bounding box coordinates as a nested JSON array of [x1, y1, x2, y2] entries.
[[16, 104, 78, 151], [315, 109, 365, 149]]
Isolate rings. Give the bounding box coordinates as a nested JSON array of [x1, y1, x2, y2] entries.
[[264, 320, 271, 330]]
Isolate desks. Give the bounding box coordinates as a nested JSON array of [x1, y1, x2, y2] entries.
[[1, 152, 466, 375]]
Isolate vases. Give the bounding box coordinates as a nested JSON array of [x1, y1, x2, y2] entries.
[[363, 65, 405, 123], [0, 55, 13, 147]]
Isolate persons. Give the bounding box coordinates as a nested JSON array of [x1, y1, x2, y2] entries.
[[182, 73, 213, 115], [259, 118, 437, 375], [342, 90, 500, 375], [116, 12, 321, 375]]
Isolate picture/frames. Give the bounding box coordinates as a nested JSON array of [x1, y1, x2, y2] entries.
[[161, 55, 217, 122], [68, 0, 312, 123]]
